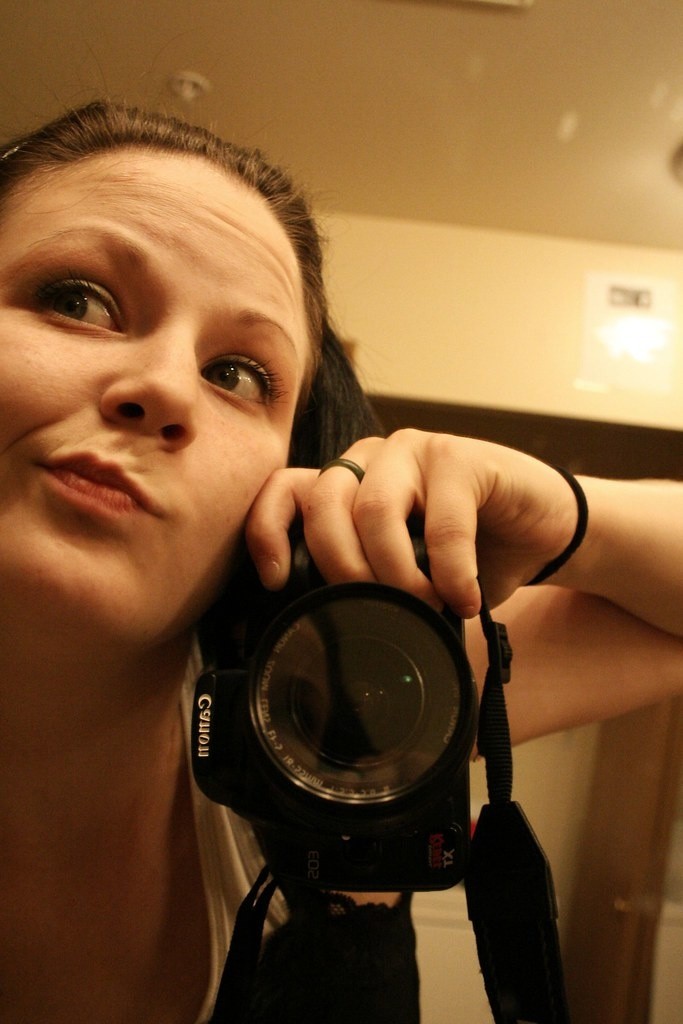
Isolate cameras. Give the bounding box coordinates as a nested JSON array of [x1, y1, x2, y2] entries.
[[190, 523, 479, 894]]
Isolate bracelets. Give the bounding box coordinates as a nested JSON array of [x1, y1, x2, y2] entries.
[[525, 466, 589, 585]]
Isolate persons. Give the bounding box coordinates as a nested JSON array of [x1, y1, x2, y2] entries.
[[0, 99, 682, 1024]]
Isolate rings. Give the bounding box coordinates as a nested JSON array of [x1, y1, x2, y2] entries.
[[319, 459, 364, 479]]
